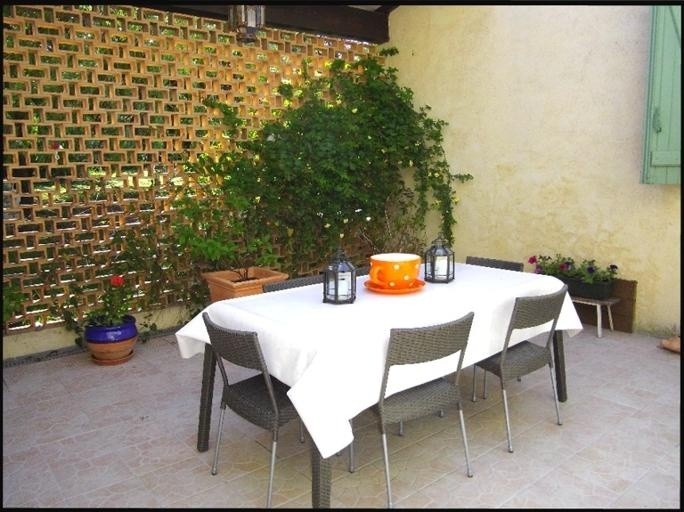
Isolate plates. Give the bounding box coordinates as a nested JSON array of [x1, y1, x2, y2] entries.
[[364, 279, 426, 295]]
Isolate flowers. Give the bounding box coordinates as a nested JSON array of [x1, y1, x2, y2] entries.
[[80, 274, 130, 326], [528, 252, 617, 283]]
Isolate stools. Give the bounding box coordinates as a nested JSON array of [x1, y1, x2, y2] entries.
[[570, 297, 619, 338]]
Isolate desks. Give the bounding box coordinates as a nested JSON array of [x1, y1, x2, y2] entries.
[[197, 260, 565, 508]]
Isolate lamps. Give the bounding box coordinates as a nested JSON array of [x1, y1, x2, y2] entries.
[[228, 5, 266, 44], [324, 246, 356, 305], [426, 233, 454, 283]]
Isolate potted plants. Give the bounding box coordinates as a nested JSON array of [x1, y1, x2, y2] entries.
[[194, 190, 288, 302]]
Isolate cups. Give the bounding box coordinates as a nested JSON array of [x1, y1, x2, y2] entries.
[[369, 252, 422, 290]]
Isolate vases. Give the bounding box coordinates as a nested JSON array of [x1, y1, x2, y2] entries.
[[564, 280, 615, 300], [83, 315, 138, 366]]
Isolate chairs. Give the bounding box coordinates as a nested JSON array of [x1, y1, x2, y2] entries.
[[369, 313, 477, 503], [473, 286, 565, 452], [378, 257, 522, 435], [202, 312, 355, 506], [262, 266, 372, 294]]
